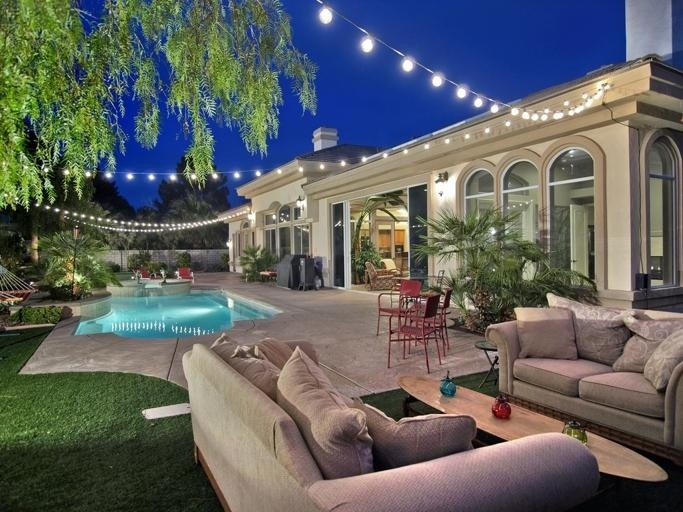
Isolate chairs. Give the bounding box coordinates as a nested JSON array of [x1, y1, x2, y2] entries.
[[388, 294, 442, 374], [365, 262, 395, 291], [150, 272, 163, 283], [408, 288, 453, 357], [140, 271, 150, 284], [381, 258, 403, 276], [178, 267, 194, 285], [377, 280, 422, 335]]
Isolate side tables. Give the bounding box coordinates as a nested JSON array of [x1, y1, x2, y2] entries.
[[475, 341, 498, 388]]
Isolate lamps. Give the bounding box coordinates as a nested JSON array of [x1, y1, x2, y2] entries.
[[598, 89, 602, 94], [434, 171, 449, 196], [579, 102, 584, 110], [521, 110, 529, 119], [247, 210, 253, 222], [558, 110, 564, 117], [361, 34, 372, 52], [403, 56, 414, 72], [594, 92, 599, 99], [568, 108, 574, 116], [588, 96, 593, 102], [457, 85, 467, 99], [541, 112, 548, 122], [432, 73, 441, 86], [575, 105, 580, 113], [491, 103, 499, 114], [510, 107, 519, 115], [553, 112, 560, 119], [295, 195, 302, 207], [585, 100, 590, 107], [474, 97, 483, 108], [319, 4, 333, 24], [531, 111, 539, 120]]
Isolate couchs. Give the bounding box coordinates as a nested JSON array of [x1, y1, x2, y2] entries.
[[486, 310, 683, 463], [181, 339, 601, 512]]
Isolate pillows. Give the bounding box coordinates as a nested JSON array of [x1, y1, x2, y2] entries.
[[276, 345, 374, 481], [514, 307, 578, 360], [353, 395, 478, 472], [256, 337, 293, 369], [546, 293, 644, 366], [210, 332, 281, 401], [612, 316, 683, 372], [644, 329, 683, 390]]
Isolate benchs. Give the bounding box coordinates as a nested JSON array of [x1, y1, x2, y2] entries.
[[260, 270, 277, 282]]
